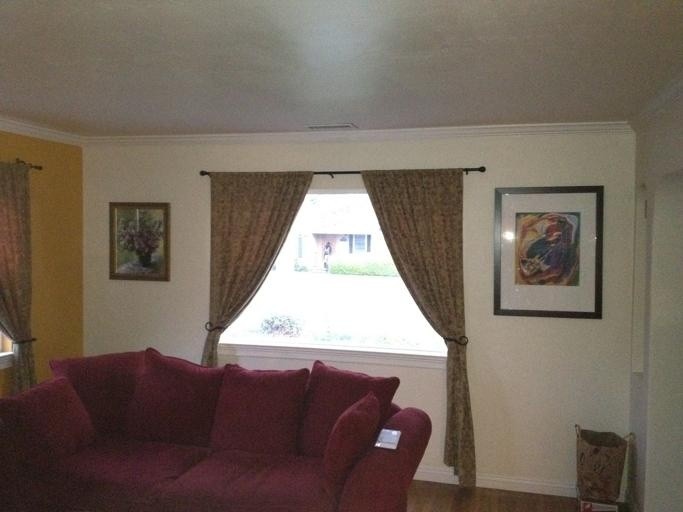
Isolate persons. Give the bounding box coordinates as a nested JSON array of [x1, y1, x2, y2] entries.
[[323, 241, 332, 271]]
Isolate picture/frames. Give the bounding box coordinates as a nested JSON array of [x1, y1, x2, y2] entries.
[[494, 186, 603, 319], [109, 202, 170, 281]]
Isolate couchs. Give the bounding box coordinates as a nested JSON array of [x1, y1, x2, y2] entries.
[[0, 398, 432, 512]]
